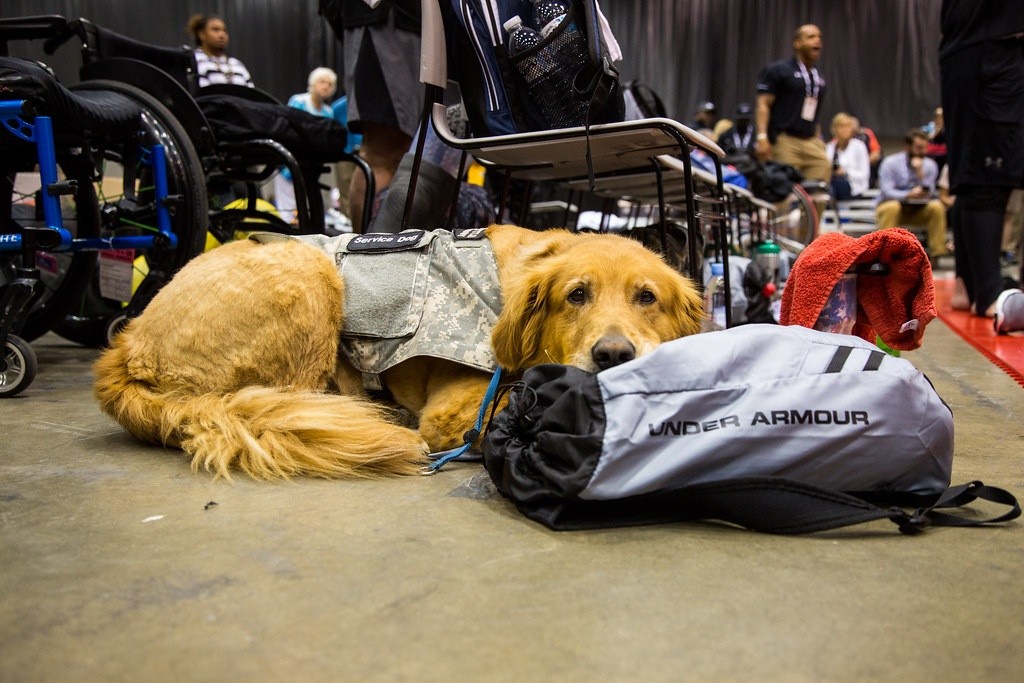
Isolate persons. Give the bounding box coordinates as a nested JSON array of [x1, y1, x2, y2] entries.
[[275, 66, 337, 230], [311, 0, 475, 234], [686, 21, 1023, 272], [188, 12, 253, 90]]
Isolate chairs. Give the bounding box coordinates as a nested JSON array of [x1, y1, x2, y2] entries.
[[400, 1, 777, 330]]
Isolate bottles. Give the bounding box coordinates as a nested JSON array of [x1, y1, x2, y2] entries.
[[703, 262, 733, 331], [752, 239, 782, 296]]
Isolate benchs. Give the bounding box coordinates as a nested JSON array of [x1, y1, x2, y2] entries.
[[821, 189, 955, 269]]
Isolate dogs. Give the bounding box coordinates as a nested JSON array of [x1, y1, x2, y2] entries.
[[93, 222, 703, 489]]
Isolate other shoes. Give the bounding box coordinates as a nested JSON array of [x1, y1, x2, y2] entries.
[[993, 289, 1024, 333], [325, 208, 352, 233]]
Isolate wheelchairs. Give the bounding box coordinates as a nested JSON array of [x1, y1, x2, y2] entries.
[[0, 14, 377, 397]]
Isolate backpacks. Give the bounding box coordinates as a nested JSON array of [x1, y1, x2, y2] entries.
[[446, 1, 627, 139]]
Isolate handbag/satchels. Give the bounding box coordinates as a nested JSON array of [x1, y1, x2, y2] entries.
[[476, 321, 1022, 540]]
